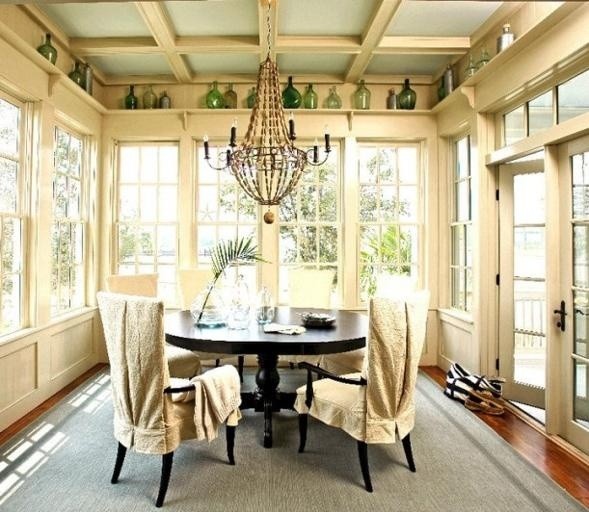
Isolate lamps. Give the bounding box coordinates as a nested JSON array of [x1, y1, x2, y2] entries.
[[203, 0, 331, 223]]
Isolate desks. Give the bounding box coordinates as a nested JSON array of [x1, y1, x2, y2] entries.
[[164, 307, 369, 448]]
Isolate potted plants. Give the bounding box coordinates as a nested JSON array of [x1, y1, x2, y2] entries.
[[189, 236, 272, 327]]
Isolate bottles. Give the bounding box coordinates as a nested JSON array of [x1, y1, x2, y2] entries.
[[159, 91, 171, 108], [354, 79, 371, 109], [206, 80, 238, 109], [282, 75, 318, 109], [37, 31, 58, 65], [463, 48, 491, 80], [436, 63, 455, 101], [247, 86, 256, 108], [68, 59, 93, 97], [496, 23, 514, 55], [327, 86, 342, 108], [143, 85, 158, 108], [125, 83, 137, 109], [189, 274, 275, 331], [388, 78, 416, 109]]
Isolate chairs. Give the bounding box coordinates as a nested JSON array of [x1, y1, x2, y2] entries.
[[277, 268, 334, 370], [294, 291, 417, 493], [179, 268, 245, 382], [96, 290, 241, 508], [324, 278, 431, 377], [106, 273, 201, 379]]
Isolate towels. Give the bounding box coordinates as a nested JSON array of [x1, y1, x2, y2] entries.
[[264, 323, 307, 335]]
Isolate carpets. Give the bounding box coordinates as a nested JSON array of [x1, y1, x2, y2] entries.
[[0, 365, 589, 512]]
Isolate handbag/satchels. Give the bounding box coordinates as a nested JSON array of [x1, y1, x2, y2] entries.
[[444, 362, 505, 406]]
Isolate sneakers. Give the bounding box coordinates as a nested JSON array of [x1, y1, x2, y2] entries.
[[468, 388, 505, 410], [465, 397, 504, 415]]
